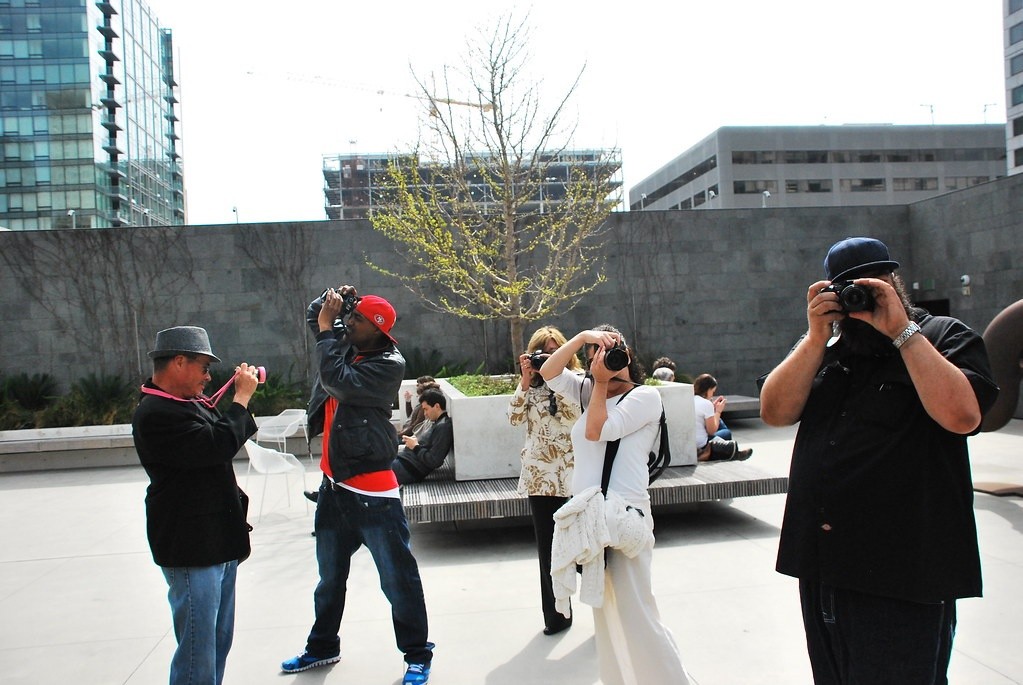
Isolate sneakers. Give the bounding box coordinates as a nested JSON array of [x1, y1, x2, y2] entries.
[[281, 649, 341, 673], [403, 661, 430, 685]]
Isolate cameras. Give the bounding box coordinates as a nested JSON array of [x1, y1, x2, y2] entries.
[[594, 340, 629, 371], [255, 366, 266, 383], [527, 350, 550, 370], [320, 287, 355, 318], [821, 280, 875, 313]]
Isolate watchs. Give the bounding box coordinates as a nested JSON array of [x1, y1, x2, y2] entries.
[[892, 320, 922, 349]]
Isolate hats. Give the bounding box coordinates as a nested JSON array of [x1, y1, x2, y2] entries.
[[825, 238, 900, 282], [147, 326, 222, 363], [356, 295, 398, 343]]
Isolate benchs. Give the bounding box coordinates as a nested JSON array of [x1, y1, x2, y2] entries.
[[708, 394, 766, 421], [400, 453, 795, 523]]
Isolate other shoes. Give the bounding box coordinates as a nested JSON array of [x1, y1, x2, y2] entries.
[[733, 448, 752, 460], [303, 491, 318, 502], [544, 617, 572, 635]]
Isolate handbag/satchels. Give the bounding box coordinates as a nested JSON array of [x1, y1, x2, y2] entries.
[[576, 547, 607, 575], [697, 444, 711, 461]]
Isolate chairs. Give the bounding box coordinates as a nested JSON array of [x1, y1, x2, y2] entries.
[[244, 408, 313, 486], [243, 437, 309, 524]]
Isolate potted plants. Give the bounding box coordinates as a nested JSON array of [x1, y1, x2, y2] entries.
[[364, 14, 697, 483]]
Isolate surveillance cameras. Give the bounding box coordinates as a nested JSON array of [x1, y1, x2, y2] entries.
[[961, 275, 970, 285]]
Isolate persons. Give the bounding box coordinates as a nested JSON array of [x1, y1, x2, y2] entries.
[[652, 357, 732, 440], [506, 325, 584, 635], [131, 325, 258, 685], [693, 374, 752, 461], [540, 324, 700, 685], [280, 285, 435, 685], [756, 237, 999, 685], [304, 377, 452, 537]]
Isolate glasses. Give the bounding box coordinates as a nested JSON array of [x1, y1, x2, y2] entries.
[[186, 355, 210, 375]]
